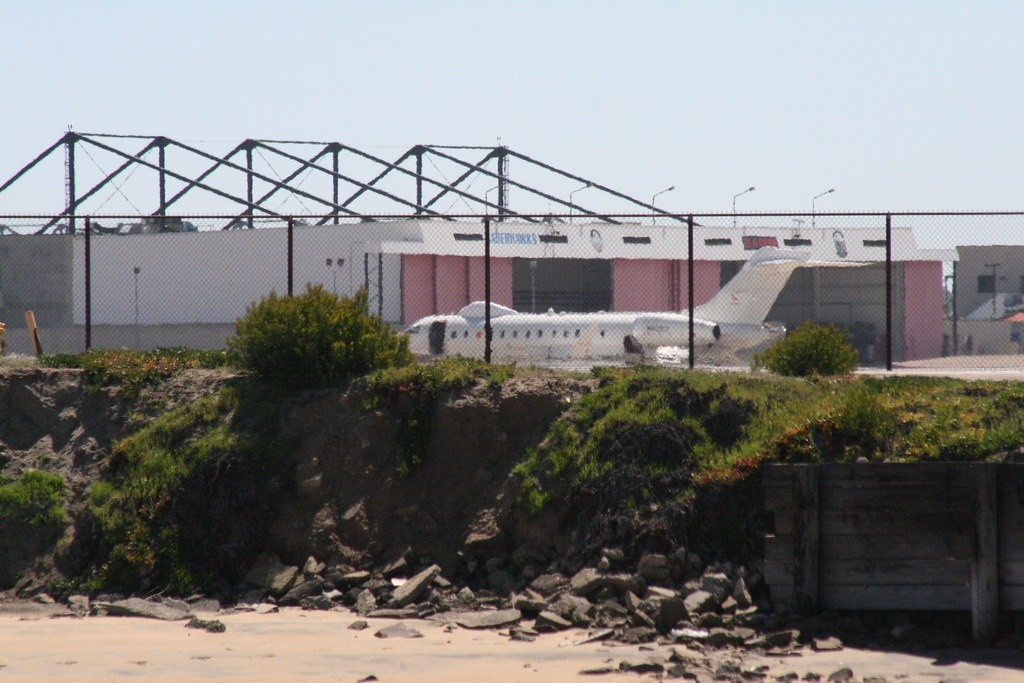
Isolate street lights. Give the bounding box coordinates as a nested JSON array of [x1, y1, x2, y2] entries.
[[652, 186, 674, 225], [733, 187, 755, 227], [569, 183, 592, 223], [812, 188, 835, 227], [326, 256, 345, 293], [985, 263, 1002, 315]]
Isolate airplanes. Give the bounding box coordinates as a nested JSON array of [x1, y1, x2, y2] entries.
[[394, 245, 815, 367]]
